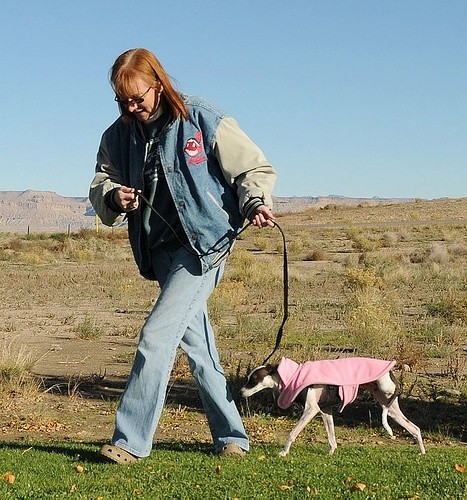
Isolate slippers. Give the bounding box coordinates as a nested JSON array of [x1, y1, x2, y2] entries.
[[218, 445, 248, 458], [98, 444, 141, 466]]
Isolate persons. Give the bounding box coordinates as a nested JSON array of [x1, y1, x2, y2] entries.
[[88, 47, 278, 464]]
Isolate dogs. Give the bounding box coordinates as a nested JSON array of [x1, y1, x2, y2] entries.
[[238, 357, 426, 457]]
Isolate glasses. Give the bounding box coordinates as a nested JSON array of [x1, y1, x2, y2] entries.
[[114, 87, 151, 104]]
